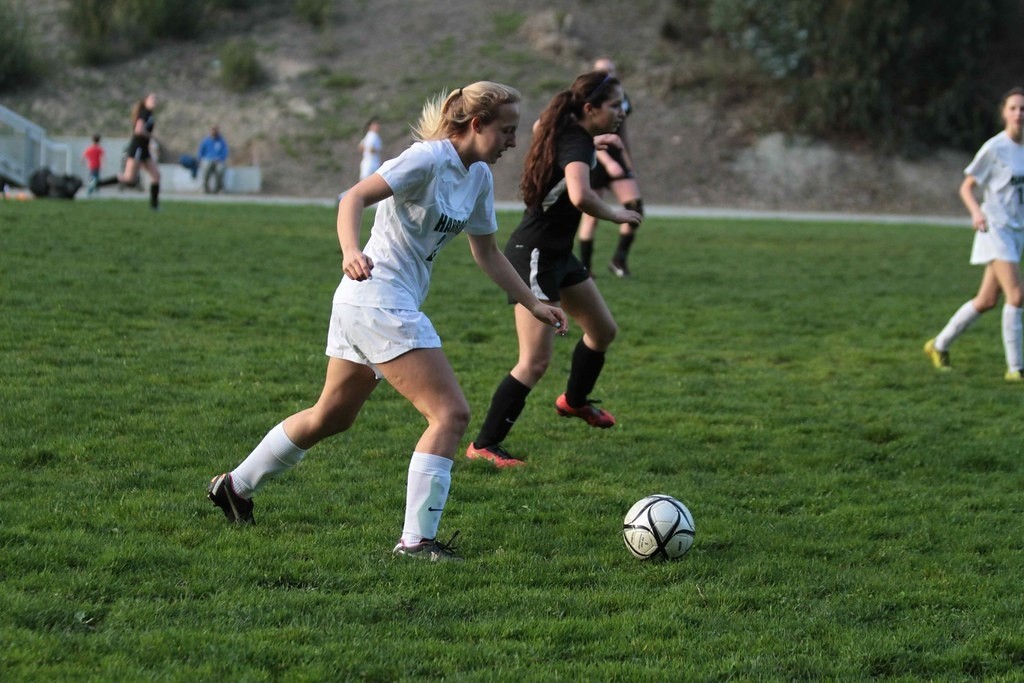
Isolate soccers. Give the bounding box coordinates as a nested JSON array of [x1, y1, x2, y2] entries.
[[622, 494, 695, 562]]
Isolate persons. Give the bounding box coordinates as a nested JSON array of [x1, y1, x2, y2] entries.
[[207, 81, 568, 561], [83, 134, 105, 194], [88, 90, 160, 209], [580, 58, 643, 276], [466, 72, 643, 468], [357, 117, 383, 180], [924, 88, 1024, 381], [199, 123, 228, 194]]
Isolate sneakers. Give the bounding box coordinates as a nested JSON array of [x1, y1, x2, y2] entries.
[[924, 340, 952, 372], [392, 529, 466, 562], [1003, 367, 1024, 381], [466, 442, 526, 468], [206, 472, 257, 527], [555, 392, 616, 429]]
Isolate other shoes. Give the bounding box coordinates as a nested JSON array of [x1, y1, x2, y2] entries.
[[607, 260, 629, 277], [586, 269, 595, 280]]
